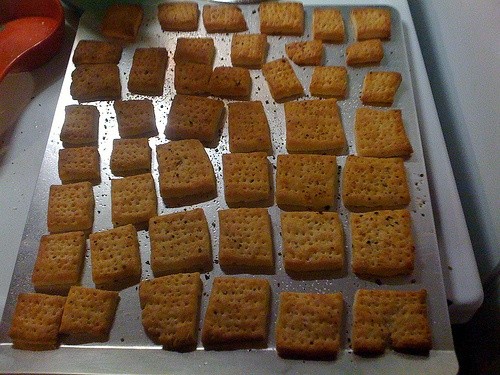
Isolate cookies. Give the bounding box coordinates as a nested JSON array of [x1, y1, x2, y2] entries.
[[9, 2, 431, 357]]
